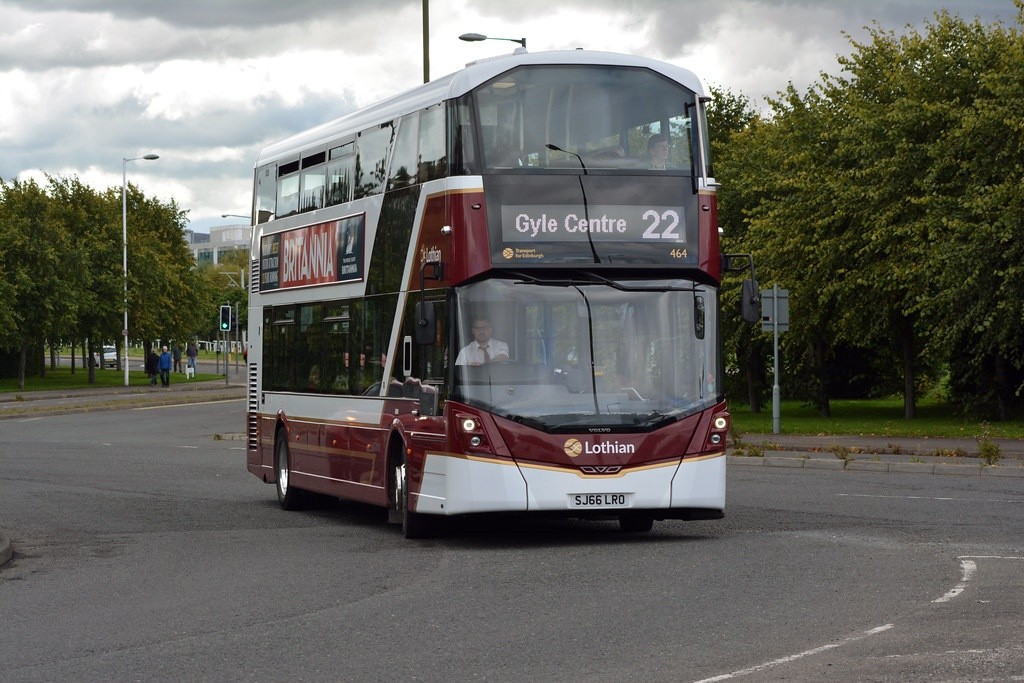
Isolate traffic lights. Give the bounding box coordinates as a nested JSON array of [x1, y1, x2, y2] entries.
[[220, 305, 231, 331]]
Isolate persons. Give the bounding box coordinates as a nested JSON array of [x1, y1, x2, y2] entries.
[[643, 134, 679, 170], [147, 342, 199, 388], [454, 315, 510, 366]]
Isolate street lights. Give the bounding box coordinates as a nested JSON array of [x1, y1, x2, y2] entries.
[[121, 153, 160, 387]]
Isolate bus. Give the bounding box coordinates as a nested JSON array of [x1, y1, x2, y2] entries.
[[244, 45, 764, 535]]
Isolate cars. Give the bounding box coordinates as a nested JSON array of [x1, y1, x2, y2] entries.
[[87, 345, 118, 367]]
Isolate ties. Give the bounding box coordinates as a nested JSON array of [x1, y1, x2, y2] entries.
[[480, 346, 490, 361]]
